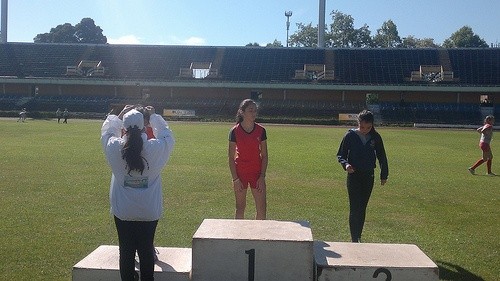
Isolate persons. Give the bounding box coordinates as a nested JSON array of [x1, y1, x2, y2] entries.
[[55, 106, 69, 124], [467, 114, 497, 176], [99, 104, 173, 281], [228, 100, 268, 220], [336, 110, 389, 242], [16, 107, 29, 122]]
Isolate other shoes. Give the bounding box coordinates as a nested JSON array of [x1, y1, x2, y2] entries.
[[468, 167, 475, 175], [489, 172, 496, 176]]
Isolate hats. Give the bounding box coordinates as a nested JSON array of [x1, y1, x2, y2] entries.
[[123, 109, 144, 131]]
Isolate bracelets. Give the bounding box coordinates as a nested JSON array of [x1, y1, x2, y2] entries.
[[232, 178, 240, 184]]
